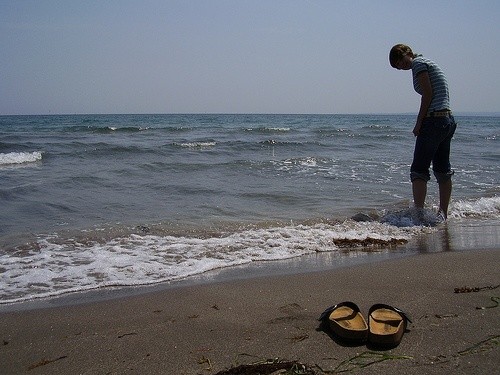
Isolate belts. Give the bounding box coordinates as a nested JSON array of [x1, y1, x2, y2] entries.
[[425, 111, 452, 117]]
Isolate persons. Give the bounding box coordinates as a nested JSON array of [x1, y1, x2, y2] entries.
[[389, 43, 457, 222]]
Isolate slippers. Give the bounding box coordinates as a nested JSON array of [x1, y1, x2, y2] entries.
[[367, 303, 413, 346], [317, 300, 369, 340]]
